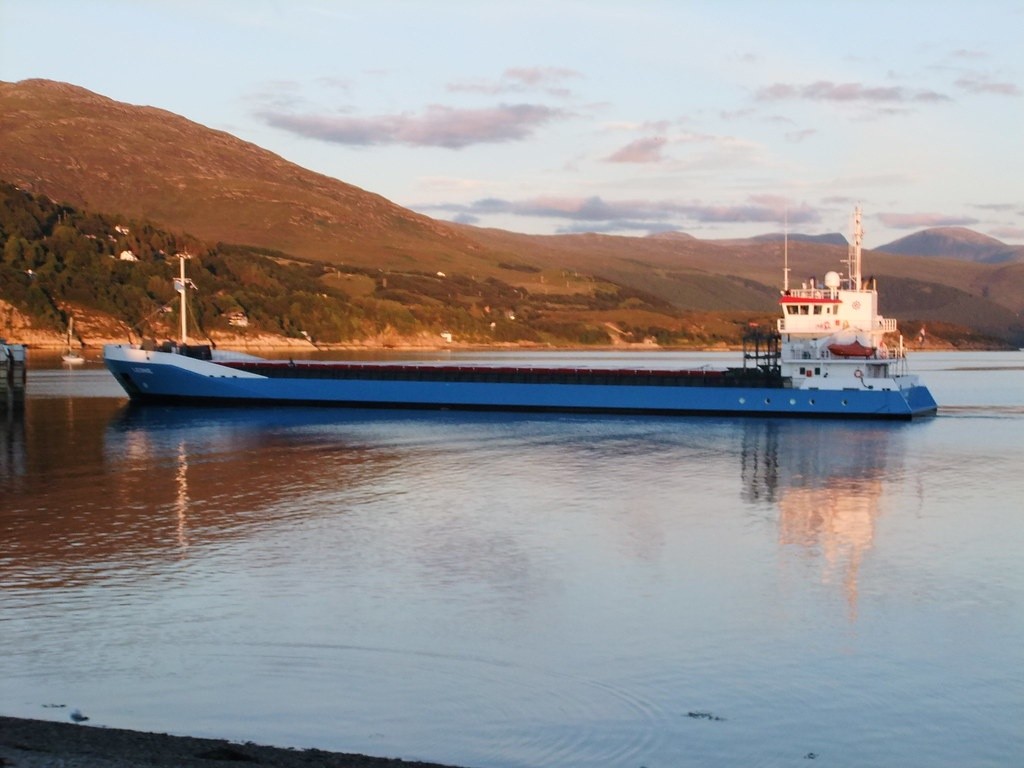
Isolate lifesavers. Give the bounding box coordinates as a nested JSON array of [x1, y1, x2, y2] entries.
[[854, 369, 864, 378]]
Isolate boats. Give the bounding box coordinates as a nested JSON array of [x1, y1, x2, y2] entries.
[[100, 205, 940, 420]]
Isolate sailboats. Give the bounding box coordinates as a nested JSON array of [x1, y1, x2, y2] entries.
[[61, 317, 86, 364]]
